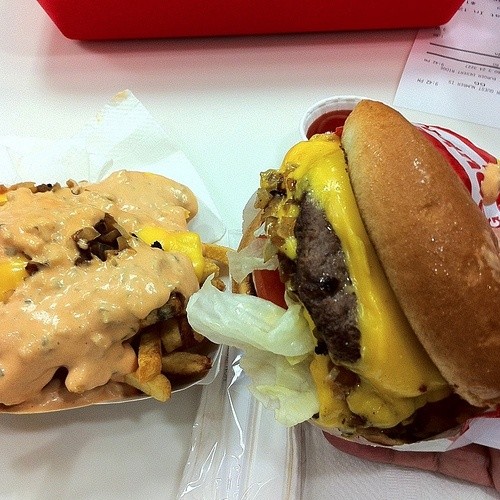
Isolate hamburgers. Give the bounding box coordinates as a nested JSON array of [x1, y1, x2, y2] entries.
[[186, 99, 499, 446]]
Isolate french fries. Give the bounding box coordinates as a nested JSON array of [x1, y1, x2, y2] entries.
[[203, 242, 235, 290], [125, 319, 211, 403]]
